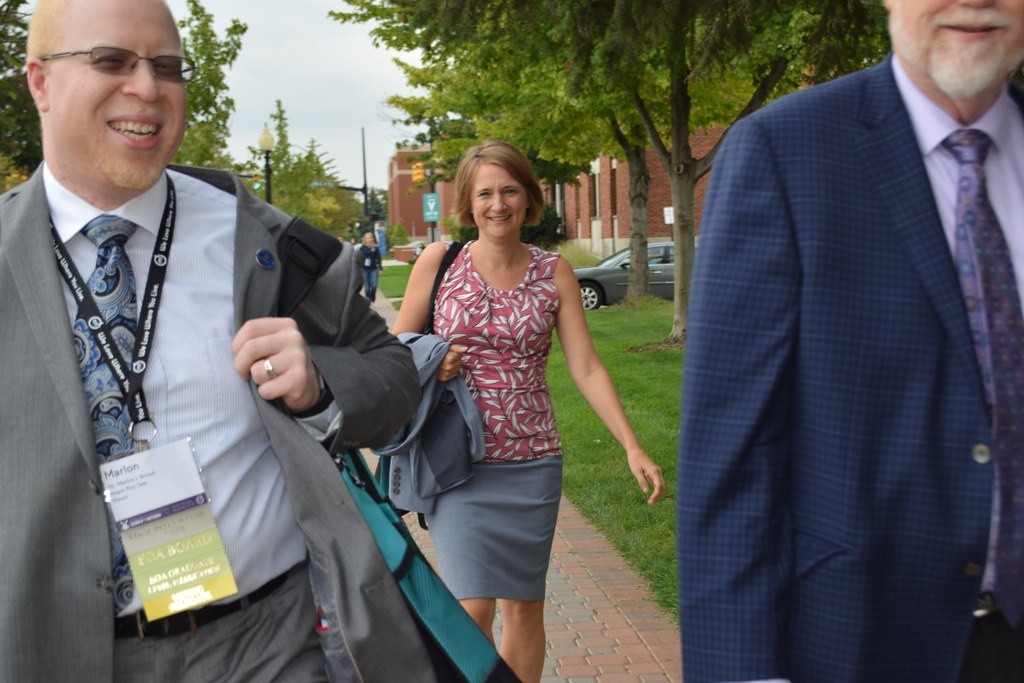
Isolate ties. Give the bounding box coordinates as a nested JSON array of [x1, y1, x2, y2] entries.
[[942, 130, 1023, 629], [67, 214, 138, 614]]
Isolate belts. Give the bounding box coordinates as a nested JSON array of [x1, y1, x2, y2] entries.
[[113, 560, 307, 638]]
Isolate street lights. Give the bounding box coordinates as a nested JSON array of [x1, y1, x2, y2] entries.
[[258, 123, 275, 205]]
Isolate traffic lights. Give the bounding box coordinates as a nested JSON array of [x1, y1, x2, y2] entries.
[[411, 162, 426, 183]]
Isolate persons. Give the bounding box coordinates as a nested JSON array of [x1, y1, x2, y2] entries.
[[675, 1, 1022, 683], [394, 143, 666, 682], [336, 233, 382, 302], [1, 2, 423, 682]]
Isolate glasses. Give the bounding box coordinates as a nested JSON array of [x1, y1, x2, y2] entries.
[[41, 46, 198, 84]]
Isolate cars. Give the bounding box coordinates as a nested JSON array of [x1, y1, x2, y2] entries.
[[571, 241, 697, 311]]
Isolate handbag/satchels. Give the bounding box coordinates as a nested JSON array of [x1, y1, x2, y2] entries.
[[328, 446, 521, 683], [373, 455, 412, 517]]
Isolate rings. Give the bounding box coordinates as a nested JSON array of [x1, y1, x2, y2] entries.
[[263, 360, 275, 378]]
